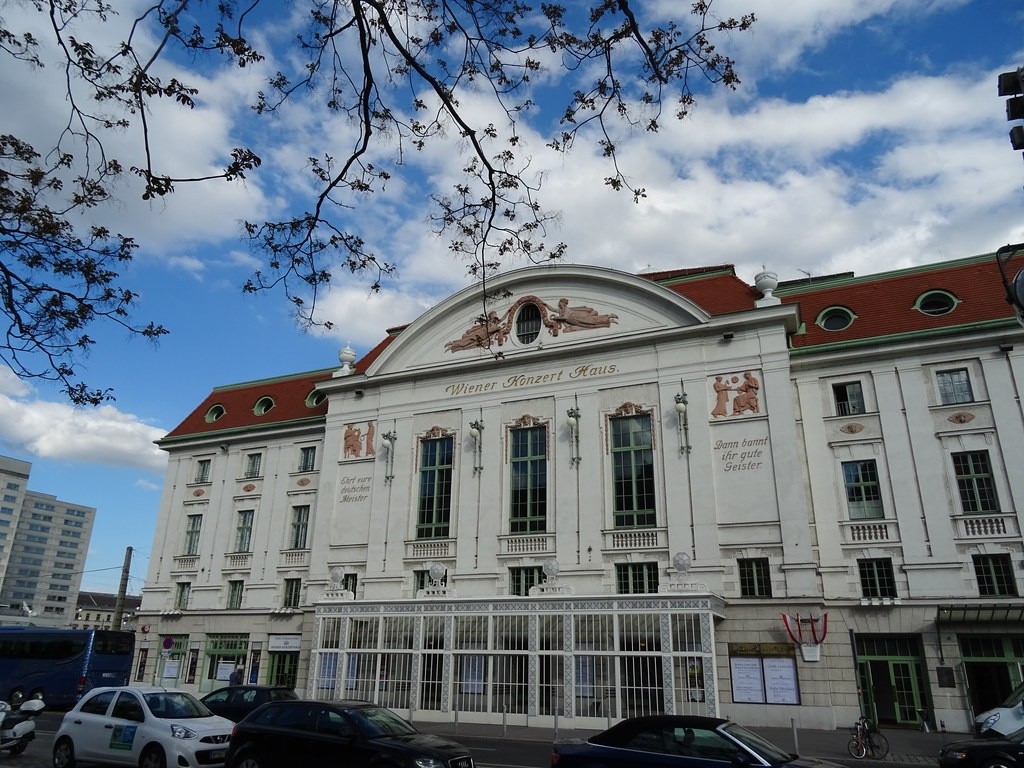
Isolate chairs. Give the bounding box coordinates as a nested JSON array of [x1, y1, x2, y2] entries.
[[118, 699, 135, 720], [682, 728, 701, 758], [664, 731, 681, 755], [148, 697, 167, 718], [309, 713, 341, 735]]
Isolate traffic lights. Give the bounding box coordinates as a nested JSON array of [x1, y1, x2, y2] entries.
[[997, 66, 1024, 151]]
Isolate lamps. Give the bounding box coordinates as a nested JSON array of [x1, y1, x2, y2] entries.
[[871, 598, 880, 605], [723, 330, 734, 339], [381, 430, 398, 483], [468, 420, 485, 475], [355, 389, 364, 397], [164, 609, 172, 615], [174, 609, 184, 615], [860, 598, 869, 606], [159, 608, 167, 615], [674, 393, 691, 454], [566, 407, 582, 466], [169, 609, 176, 614], [221, 444, 229, 452], [893, 597, 903, 605], [883, 598, 891, 605], [269, 607, 295, 614]]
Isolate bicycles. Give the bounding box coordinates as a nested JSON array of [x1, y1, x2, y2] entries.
[[847, 716, 890, 760]]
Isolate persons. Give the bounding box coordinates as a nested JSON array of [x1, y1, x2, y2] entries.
[[847, 425, 856, 432], [519, 415, 531, 427], [196, 490, 201, 496], [959, 413, 965, 422], [624, 402, 633, 413], [344, 420, 375, 457], [431, 425, 440, 437], [711, 371, 759, 418], [445, 311, 509, 353], [246, 485, 252, 491], [229, 668, 240, 686], [300, 479, 307, 485], [542, 298, 619, 332]]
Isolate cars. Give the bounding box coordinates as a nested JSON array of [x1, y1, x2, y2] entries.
[[52, 687, 237, 768], [551, 714, 848, 768], [936, 726, 1024, 768], [175, 684, 301, 722], [972, 680, 1024, 740], [227, 699, 476, 768]]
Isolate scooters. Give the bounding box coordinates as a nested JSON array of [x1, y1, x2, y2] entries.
[[0, 699, 47, 756]]
